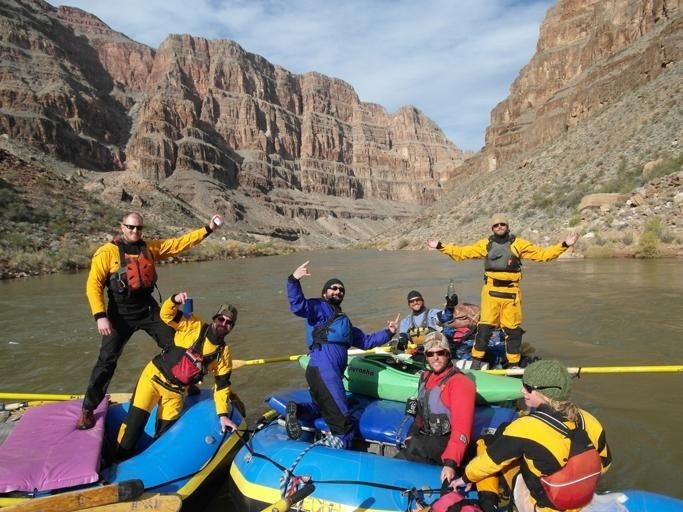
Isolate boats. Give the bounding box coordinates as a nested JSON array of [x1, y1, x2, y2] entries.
[[288, 347, 534, 404], [0, 382, 250, 512], [266, 382, 537, 443], [228, 416, 682, 512]]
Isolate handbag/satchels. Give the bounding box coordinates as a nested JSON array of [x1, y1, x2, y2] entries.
[[443, 303, 505, 360]]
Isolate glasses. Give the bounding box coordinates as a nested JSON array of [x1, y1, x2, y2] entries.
[[123, 224, 143, 230], [329, 287, 344, 292], [425, 349, 446, 356], [524, 383, 562, 394], [408, 298, 422, 303], [492, 224, 506, 227], [218, 318, 233, 326]]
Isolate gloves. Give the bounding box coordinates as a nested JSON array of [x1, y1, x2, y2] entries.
[[445, 293, 459, 308]]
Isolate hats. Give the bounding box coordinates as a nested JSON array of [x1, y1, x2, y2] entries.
[[424, 331, 453, 360], [322, 279, 345, 298], [407, 290, 423, 301], [212, 303, 238, 327], [523, 359, 573, 401], [491, 213, 509, 231]]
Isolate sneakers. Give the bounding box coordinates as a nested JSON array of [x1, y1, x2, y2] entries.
[[285, 401, 303, 440], [77, 405, 94, 429]]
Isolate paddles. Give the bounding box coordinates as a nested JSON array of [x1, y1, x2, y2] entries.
[[232, 346, 392, 369]]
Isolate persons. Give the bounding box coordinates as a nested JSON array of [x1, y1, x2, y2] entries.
[[114, 292, 239, 455], [427, 214, 579, 371], [393, 331, 476, 484], [397, 290, 458, 352], [78, 211, 224, 430], [448, 359, 613, 512], [287, 259, 400, 449]]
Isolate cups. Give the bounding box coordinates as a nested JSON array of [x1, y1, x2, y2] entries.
[[183, 297, 193, 319]]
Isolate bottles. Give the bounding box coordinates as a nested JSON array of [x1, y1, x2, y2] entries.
[[447, 280, 455, 301]]
